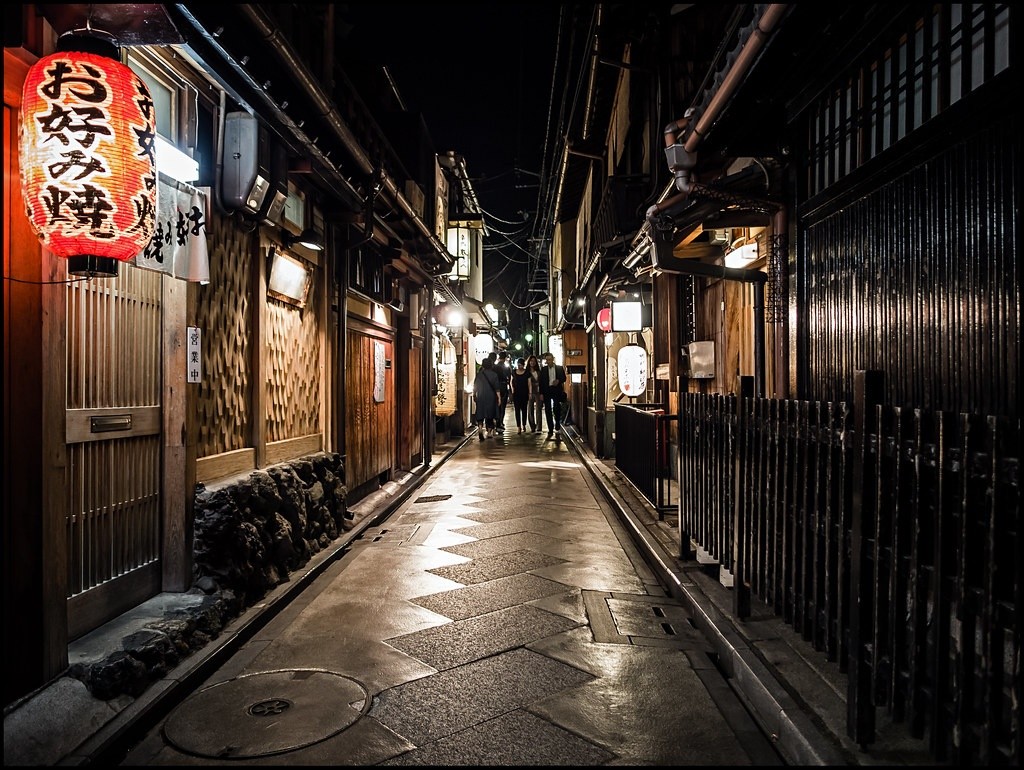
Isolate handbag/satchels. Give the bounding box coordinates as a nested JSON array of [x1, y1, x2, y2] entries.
[[559, 392, 567, 402]]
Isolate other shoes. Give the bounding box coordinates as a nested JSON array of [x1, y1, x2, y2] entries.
[[496, 425, 506, 430], [546, 432, 553, 440], [479, 431, 484, 440], [531, 424, 536, 432], [487, 434, 493, 438], [537, 431, 543, 434], [517, 427, 521, 435], [555, 433, 562, 441], [523, 425, 526, 432]]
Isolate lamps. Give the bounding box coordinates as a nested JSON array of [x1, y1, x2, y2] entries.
[[610, 293, 644, 334], [490, 320, 508, 343], [280, 227, 324, 253], [723, 242, 759, 270], [687, 340, 716, 381]]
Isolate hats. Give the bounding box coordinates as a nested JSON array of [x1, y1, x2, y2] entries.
[[499, 352, 505, 359]]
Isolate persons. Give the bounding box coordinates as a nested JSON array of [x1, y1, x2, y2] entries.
[[473, 352, 566, 440]]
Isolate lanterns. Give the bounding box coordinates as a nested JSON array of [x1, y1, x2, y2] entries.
[[617, 343, 648, 398], [17, 27, 156, 278], [597, 308, 612, 332], [429, 346, 457, 418], [548, 334, 563, 367], [473, 333, 494, 366]]
